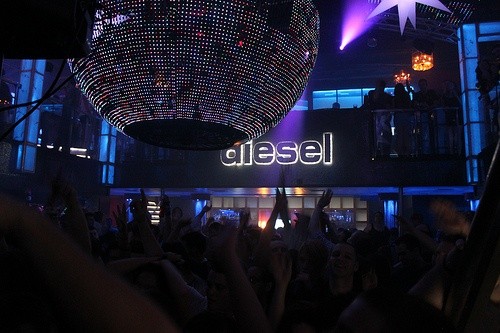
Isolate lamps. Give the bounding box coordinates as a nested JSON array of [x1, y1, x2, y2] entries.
[[411, 48, 435, 71]]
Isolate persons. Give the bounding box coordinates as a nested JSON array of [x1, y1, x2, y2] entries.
[[0, 75, 498, 331]]
